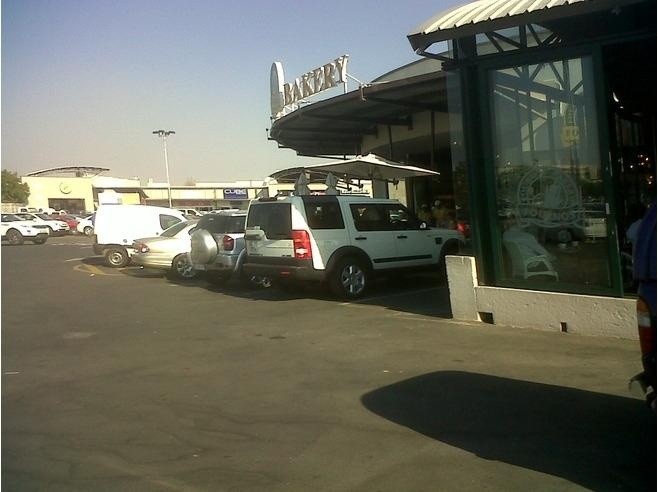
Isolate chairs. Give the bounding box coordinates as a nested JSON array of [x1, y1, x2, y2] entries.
[[502, 239, 559, 283]]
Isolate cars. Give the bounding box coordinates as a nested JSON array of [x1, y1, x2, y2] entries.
[[130, 216, 200, 281], [2, 206, 97, 244], [498, 202, 608, 242], [176, 206, 248, 221]]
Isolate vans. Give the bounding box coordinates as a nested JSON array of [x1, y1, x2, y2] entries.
[[244, 195, 470, 300]]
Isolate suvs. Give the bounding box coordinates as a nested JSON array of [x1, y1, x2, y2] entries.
[[190, 208, 248, 290]]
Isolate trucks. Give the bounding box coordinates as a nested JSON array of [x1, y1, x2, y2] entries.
[[94, 204, 195, 268]]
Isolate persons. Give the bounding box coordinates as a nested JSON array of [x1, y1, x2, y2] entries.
[[431, 200, 448, 227], [502, 215, 560, 278], [418, 204, 431, 225]]
